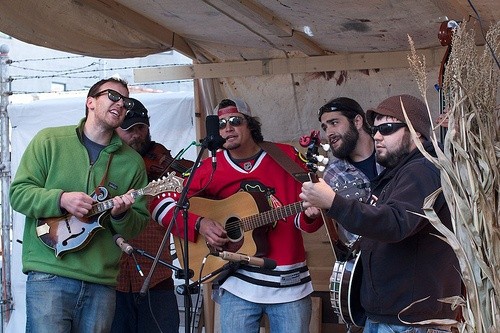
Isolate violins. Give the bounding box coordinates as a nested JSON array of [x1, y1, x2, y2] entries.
[[140, 143, 195, 182]]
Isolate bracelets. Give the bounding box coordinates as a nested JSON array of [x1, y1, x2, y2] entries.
[[195, 216, 205, 233]]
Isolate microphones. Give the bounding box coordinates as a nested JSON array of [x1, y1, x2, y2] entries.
[[112, 234, 134, 256], [212, 250, 276, 269], [206, 115, 221, 171]]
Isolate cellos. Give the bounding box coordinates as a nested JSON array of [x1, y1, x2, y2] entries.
[[437, 20, 459, 143]]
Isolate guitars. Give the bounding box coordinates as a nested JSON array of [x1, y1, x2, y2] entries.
[[36, 170, 185, 258], [173, 177, 371, 284], [299, 130, 368, 328]]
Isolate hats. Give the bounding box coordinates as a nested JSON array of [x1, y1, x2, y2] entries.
[[213, 97, 252, 117], [119, 98, 150, 130], [366, 95, 431, 140]]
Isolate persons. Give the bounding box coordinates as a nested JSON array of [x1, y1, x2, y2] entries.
[[111, 96, 180, 333], [317, 95, 388, 246], [297, 94, 461, 333], [150, 95, 324, 333], [9, 77, 153, 333]]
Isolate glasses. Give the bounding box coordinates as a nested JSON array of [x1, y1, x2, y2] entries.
[[92, 89, 135, 110], [318, 104, 359, 121], [219, 116, 248, 129], [372, 123, 409, 135]]
